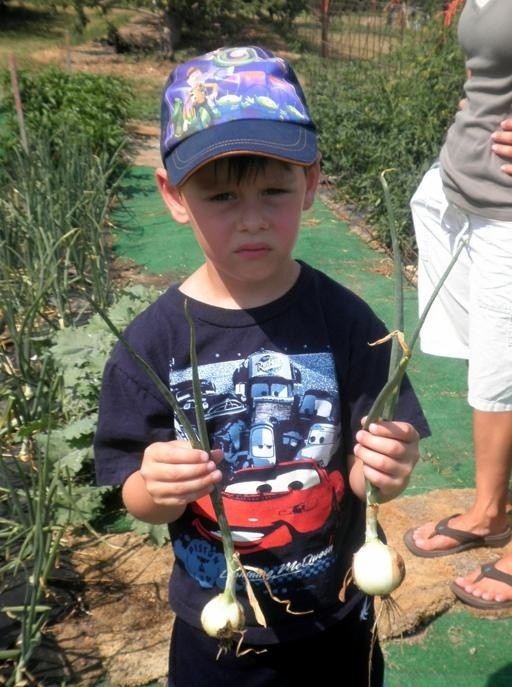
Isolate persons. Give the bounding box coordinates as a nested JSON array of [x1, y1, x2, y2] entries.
[[408, 0, 511, 609], [90, 44, 432, 687]]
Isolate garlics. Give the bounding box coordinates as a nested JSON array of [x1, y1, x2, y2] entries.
[[353, 541, 407, 598], [200, 595, 246, 637]]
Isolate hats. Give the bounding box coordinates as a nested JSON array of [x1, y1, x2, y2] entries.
[[159, 45, 321, 190]]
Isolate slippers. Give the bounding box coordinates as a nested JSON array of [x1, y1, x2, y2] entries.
[[450, 560, 512, 611], [402, 512, 512, 559]]
